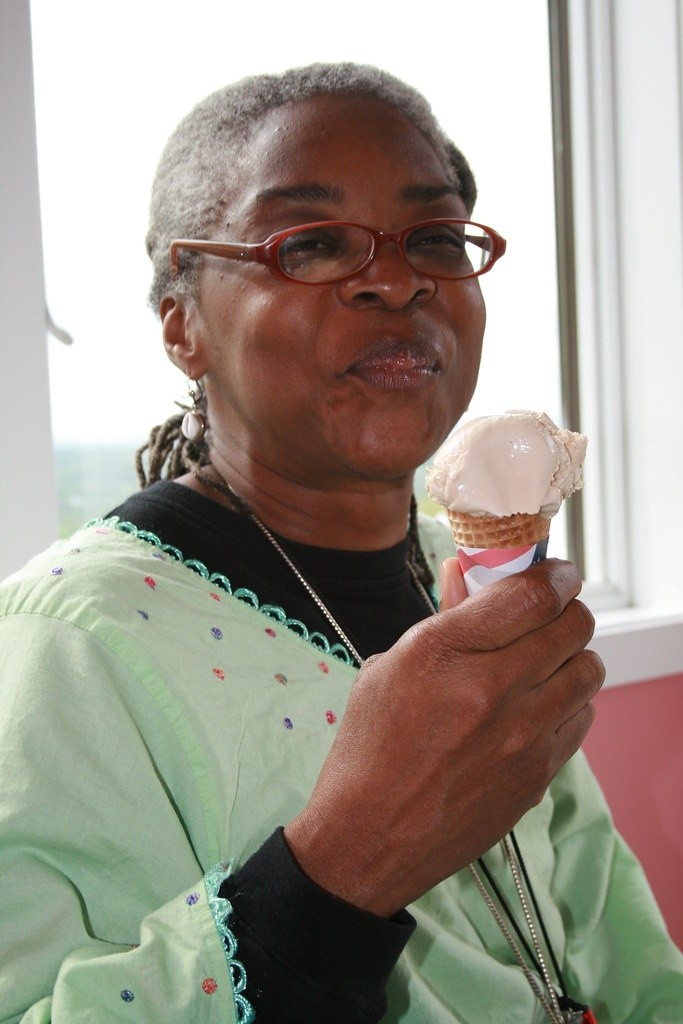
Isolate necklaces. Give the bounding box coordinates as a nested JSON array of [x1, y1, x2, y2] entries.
[[202, 450, 598, 1024]]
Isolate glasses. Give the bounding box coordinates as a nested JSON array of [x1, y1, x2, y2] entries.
[[170, 217, 506, 286]]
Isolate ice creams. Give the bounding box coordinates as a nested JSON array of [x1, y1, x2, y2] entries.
[[425, 409, 589, 549]]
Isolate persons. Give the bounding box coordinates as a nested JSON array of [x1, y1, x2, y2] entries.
[[1, 62, 683, 1024]]
